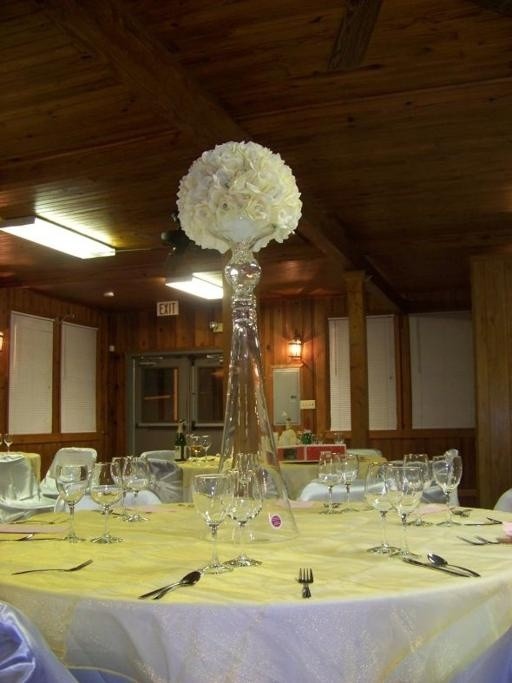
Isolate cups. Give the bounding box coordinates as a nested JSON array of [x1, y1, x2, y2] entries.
[[299, 431, 345, 444]]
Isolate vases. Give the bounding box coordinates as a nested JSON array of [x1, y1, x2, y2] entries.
[[212, 247, 299, 546]]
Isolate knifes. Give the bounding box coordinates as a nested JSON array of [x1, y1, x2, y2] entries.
[[403, 558, 466, 579]]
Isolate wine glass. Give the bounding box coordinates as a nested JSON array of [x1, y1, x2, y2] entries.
[[0, 431, 16, 456], [317, 448, 358, 516], [221, 467, 267, 570], [191, 475, 236, 575], [54, 454, 151, 547], [362, 452, 464, 559], [186, 433, 212, 464]]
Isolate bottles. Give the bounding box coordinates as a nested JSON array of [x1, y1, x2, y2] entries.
[[173, 418, 187, 461]]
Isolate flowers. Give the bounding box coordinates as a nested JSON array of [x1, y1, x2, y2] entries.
[[175, 141, 304, 253]]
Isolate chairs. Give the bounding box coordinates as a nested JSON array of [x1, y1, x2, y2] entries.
[[493, 488, 512, 513], [139, 450, 180, 462], [457, 629, 512, 683], [296, 470, 460, 506], [0, 600, 137, 683], [54, 485, 161, 512], [146, 458, 183, 503], [39, 447, 97, 500], [12, 451, 41, 484]]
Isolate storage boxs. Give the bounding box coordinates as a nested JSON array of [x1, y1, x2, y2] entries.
[[275, 445, 346, 463]]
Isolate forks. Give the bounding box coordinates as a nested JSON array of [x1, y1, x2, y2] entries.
[[10, 557, 94, 577], [0, 531, 34, 542], [455, 534, 504, 546], [296, 565, 315, 597]]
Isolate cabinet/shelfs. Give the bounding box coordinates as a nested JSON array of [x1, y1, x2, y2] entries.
[[0, 289, 109, 444], [312, 297, 477, 439]]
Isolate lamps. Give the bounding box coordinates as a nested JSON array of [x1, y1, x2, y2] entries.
[[0, 214, 116, 260], [286, 328, 302, 360], [164, 273, 225, 300]]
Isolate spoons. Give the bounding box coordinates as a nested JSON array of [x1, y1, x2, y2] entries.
[[140, 569, 200, 600], [424, 549, 483, 578]]
